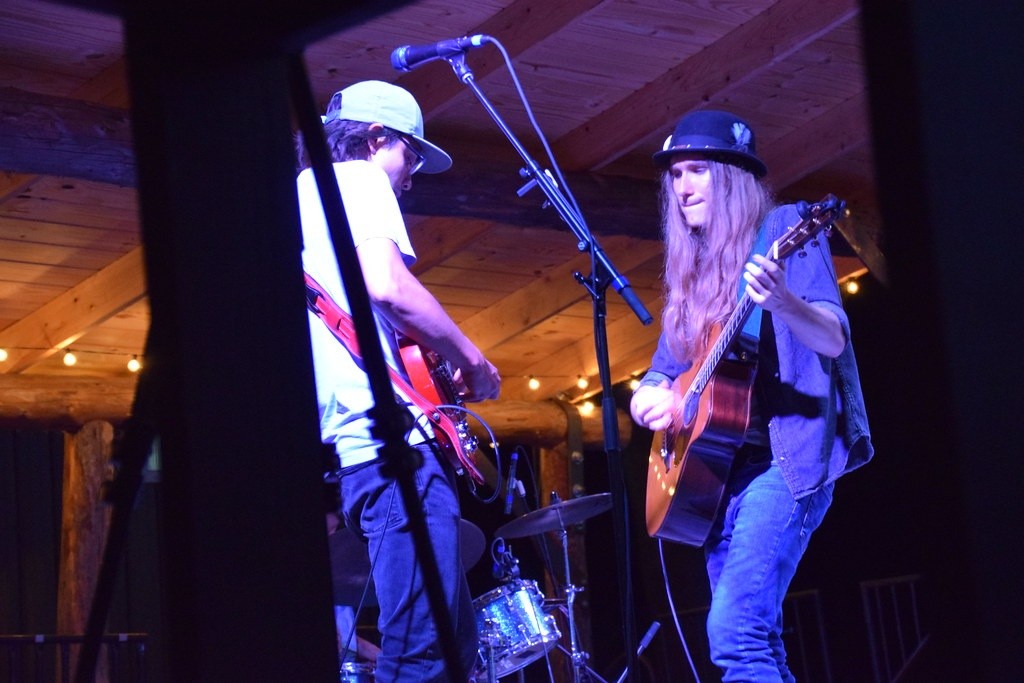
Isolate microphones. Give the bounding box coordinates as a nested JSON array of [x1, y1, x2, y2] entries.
[[390, 35, 490, 74], [504, 453, 518, 514]]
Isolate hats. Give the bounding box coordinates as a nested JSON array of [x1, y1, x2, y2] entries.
[[651, 111, 768, 179], [319, 80, 453, 174]]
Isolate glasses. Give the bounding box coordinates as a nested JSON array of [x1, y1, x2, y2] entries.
[[384, 128, 426, 174]]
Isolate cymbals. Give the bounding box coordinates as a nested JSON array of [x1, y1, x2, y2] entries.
[[329, 518, 486, 610], [494, 492, 613, 539]]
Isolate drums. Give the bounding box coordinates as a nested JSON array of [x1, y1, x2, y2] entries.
[[339, 662, 375, 683], [468, 579, 559, 683]]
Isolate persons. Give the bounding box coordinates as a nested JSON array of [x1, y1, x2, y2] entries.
[[296, 80, 502, 683], [629, 111, 874, 681]]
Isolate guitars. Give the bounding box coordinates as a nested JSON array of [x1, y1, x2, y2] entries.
[[396, 336, 477, 476], [644, 191, 847, 549]]
